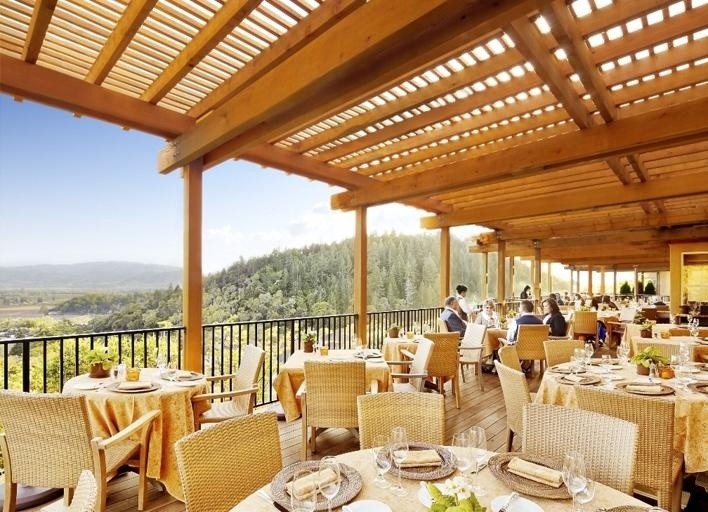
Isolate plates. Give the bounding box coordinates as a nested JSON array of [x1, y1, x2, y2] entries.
[[390, 427, 409, 497], [548, 360, 708, 397], [488, 450, 583, 501], [271, 461, 364, 509], [340, 500, 392, 512], [105, 380, 162, 393], [157, 371, 202, 381], [492, 496, 544, 511], [418, 484, 478, 507], [355, 353, 379, 358], [377, 446, 459, 481], [73, 381, 104, 390]]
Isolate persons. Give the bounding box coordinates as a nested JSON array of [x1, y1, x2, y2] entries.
[[621, 280, 656, 294], [444, 282, 587, 372]]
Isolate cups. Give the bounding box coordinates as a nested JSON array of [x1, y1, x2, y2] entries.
[[292, 470, 318, 511]]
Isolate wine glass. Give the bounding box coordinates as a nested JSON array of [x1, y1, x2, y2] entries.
[[562, 448, 594, 512], [450, 434, 472, 490], [571, 313, 701, 389], [471, 427, 489, 495], [319, 455, 341, 512], [370, 434, 390, 489], [156, 349, 168, 371]]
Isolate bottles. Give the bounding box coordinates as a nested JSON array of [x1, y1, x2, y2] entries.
[[114, 366, 118, 378], [313, 345, 316, 354], [120, 361, 126, 376]]
